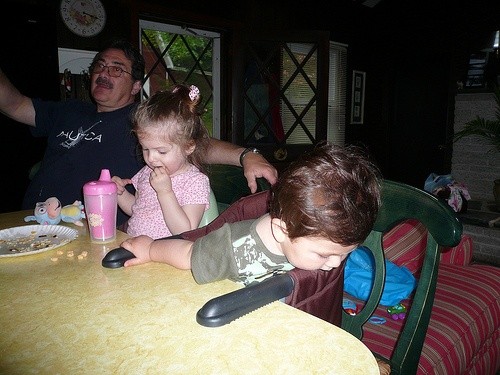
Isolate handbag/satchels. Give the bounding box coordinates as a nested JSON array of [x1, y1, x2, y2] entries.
[[343, 246, 418, 306]]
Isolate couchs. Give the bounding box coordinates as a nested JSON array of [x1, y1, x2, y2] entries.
[[342, 217, 500, 375]]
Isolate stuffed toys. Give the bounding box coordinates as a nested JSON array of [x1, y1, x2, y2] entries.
[[24, 197, 86, 227]]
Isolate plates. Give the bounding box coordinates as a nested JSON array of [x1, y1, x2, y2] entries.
[[0, 225, 79, 257]]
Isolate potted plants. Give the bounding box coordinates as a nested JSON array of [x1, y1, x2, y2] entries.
[[449, 78, 500, 210]]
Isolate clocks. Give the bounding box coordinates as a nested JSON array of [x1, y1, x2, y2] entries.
[[61, 0, 106, 38]]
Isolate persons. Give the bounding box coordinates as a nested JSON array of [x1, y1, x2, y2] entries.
[[112, 85, 209, 241], [0, 41, 279, 230], [119, 142, 382, 305]]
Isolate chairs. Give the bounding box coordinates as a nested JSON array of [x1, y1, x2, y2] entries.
[[340, 180, 463, 375], [210, 168, 267, 218]]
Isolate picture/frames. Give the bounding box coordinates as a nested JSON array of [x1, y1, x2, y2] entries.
[[350, 70, 367, 124]]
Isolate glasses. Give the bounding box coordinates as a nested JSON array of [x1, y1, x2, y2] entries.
[[92, 62, 133, 77]]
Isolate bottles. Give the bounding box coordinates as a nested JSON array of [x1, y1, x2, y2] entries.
[[83, 169, 118, 244]]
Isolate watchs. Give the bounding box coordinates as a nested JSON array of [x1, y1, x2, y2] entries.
[[240, 146, 260, 166]]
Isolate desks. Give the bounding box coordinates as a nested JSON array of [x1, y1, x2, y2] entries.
[[0, 206, 379, 375]]
